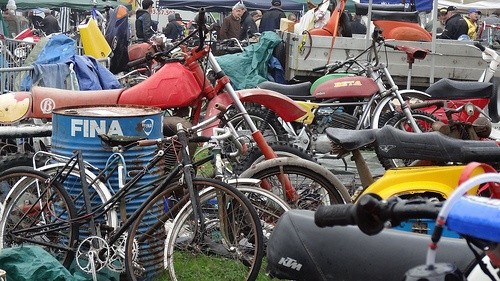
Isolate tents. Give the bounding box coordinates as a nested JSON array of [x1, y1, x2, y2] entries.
[[436, 0, 500, 17], [294, 0, 357, 16], [160, 1, 305, 31], [0, 0, 132, 36]]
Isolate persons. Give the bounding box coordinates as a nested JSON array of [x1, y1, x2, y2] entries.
[[164, 13, 186, 41], [436, 8, 448, 39], [259, 0, 286, 33], [483, 7, 500, 46], [219, 3, 246, 40], [464, 7, 482, 40], [439, 6, 468, 40], [286, 14, 297, 22], [348, 13, 367, 34], [41, 8, 62, 34], [239, 9, 263, 42], [135, 0, 158, 43]]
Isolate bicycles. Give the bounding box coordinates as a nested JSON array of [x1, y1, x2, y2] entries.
[[0, 0, 500, 281]]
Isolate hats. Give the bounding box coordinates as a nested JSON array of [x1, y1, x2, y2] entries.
[[439, 8, 448, 12], [447, 6, 457, 11], [468, 8, 480, 13], [272, 0, 281, 6]]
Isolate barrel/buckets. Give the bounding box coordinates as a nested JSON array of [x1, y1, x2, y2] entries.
[[50, 103, 166, 281]]
